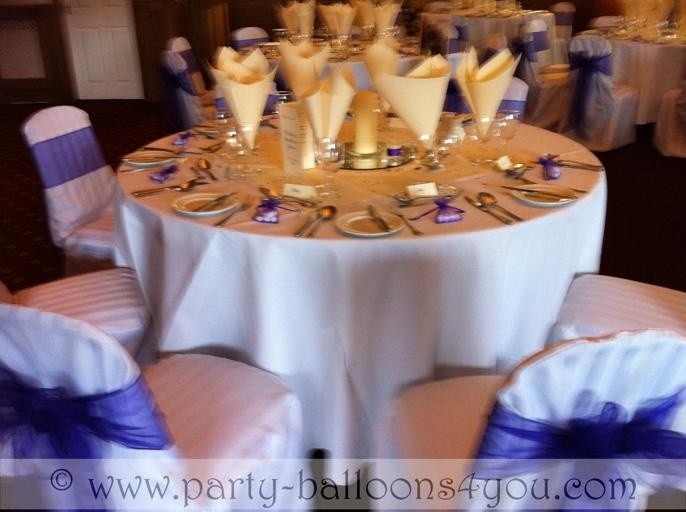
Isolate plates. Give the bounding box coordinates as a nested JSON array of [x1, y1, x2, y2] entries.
[[196, 124, 227, 137], [510, 184, 573, 207], [122, 152, 177, 166], [336, 210, 403, 237], [171, 190, 240, 215]]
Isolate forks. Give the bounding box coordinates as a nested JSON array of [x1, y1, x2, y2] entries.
[[215, 193, 255, 227]]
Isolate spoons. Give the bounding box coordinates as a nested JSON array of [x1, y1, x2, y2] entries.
[[197, 157, 218, 183], [258, 186, 319, 207], [391, 192, 455, 202], [291, 206, 337, 239], [480, 190, 524, 222], [134, 179, 201, 201]]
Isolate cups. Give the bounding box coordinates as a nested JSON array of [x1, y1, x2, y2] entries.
[[270, 90, 294, 111], [449, 0, 524, 19], [269, 26, 401, 59], [617, 15, 680, 43], [211, 107, 235, 129], [440, 116, 465, 147]]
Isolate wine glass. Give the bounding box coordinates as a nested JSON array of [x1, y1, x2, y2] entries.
[[221, 126, 263, 181], [312, 137, 345, 200], [408, 134, 434, 183], [370, 105, 386, 154], [471, 113, 494, 166], [495, 108, 518, 163], [435, 134, 462, 194]]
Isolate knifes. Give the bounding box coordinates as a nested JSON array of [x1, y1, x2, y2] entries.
[[464, 193, 511, 227], [117, 156, 186, 162], [501, 183, 574, 203], [187, 191, 242, 211], [368, 201, 390, 231], [142, 147, 205, 156]]
